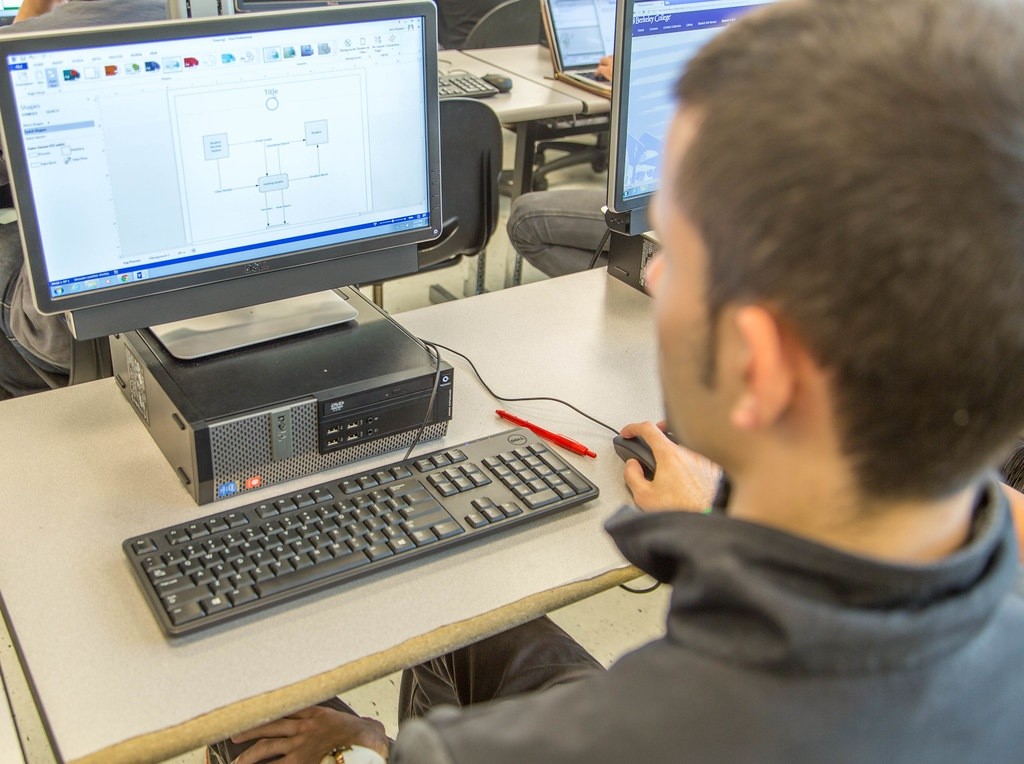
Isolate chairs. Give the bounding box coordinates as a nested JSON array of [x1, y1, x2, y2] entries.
[[356, 96, 505, 306], [463, 0, 547, 49], [533, 131, 609, 188]]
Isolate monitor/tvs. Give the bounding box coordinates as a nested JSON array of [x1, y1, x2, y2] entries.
[[0, 0, 23, 26], [0, 0, 444, 359], [235, 0, 370, 13], [604, 0, 780, 235]]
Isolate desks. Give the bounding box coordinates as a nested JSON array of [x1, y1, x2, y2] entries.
[[0, 266, 663, 764], [0, 41, 611, 300]]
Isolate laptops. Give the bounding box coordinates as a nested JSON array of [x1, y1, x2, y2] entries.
[[541, 0, 617, 99]]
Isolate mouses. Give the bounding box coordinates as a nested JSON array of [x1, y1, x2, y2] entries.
[[481, 73, 513, 92], [612, 432, 680, 483]]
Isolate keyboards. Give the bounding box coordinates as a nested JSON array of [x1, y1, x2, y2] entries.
[[121, 427, 600, 638], [438, 74, 500, 99]]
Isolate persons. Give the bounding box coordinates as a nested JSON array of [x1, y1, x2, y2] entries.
[[0, 0, 181, 389], [210, 0, 1024, 763], [504, 50, 619, 277]]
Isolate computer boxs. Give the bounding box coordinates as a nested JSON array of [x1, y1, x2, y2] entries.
[[607, 230, 660, 298], [107, 283, 454, 506]]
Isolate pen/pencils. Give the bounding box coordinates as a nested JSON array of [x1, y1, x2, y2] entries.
[[494, 409, 600, 458]]
